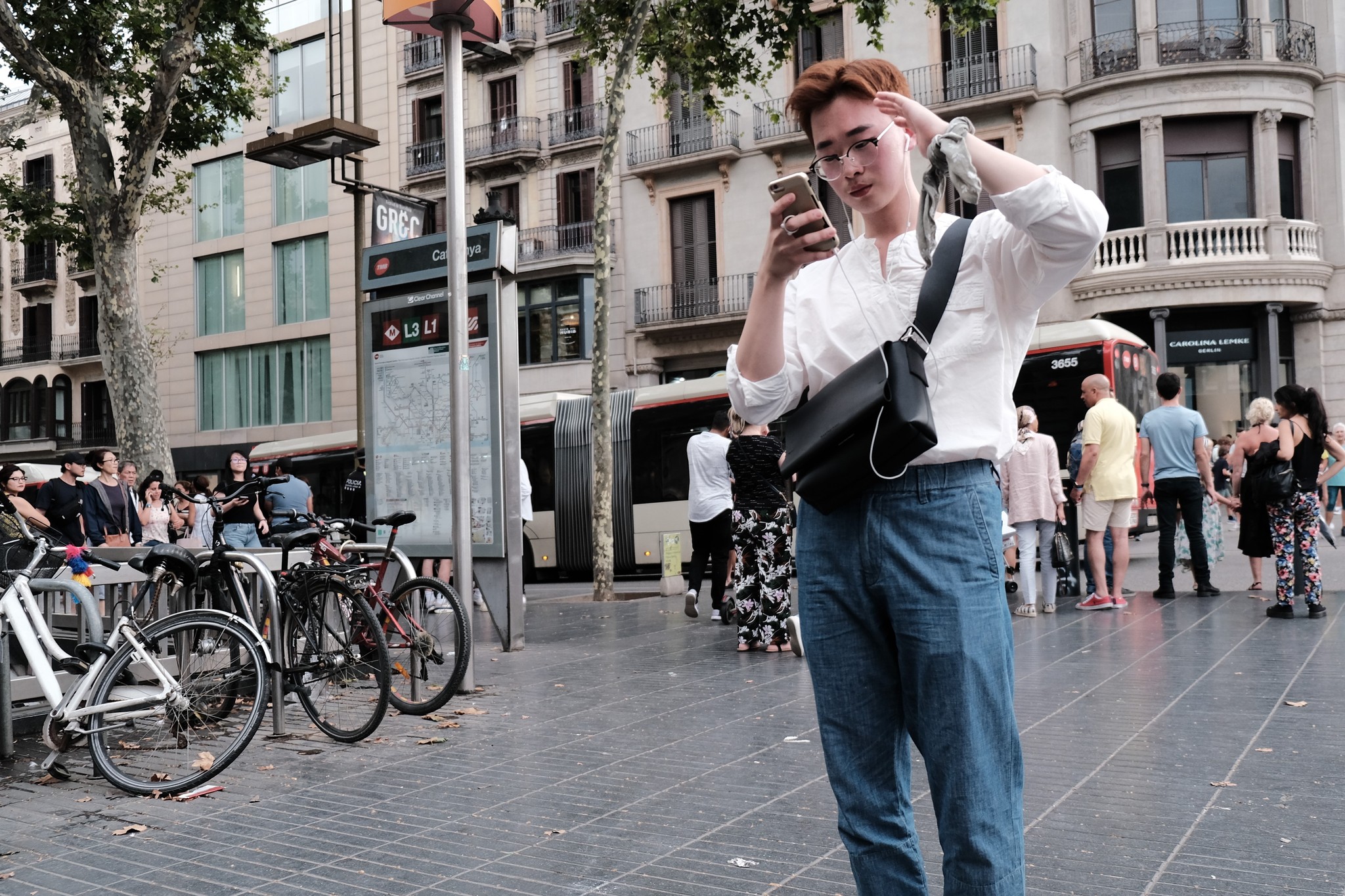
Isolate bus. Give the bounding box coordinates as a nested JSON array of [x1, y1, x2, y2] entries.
[[248, 321, 1164, 585], [1, 464, 138, 506]]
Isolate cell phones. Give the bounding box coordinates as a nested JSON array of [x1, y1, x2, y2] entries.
[[238, 496, 249, 503], [149, 492, 152, 497], [768, 172, 840, 253]]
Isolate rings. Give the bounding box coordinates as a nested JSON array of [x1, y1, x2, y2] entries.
[[781, 215, 800, 236]]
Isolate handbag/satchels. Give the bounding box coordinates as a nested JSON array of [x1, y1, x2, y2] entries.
[[102, 521, 131, 547], [1252, 417, 1295, 499], [1051, 516, 1074, 569], [781, 219, 974, 516], [786, 500, 797, 529], [177, 526, 201, 547]]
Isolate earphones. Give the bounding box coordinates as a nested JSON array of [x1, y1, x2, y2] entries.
[[905, 133, 910, 152]]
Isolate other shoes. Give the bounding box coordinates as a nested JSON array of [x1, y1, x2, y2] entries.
[[425, 592, 526, 613], [1016, 602, 1056, 617], [710, 610, 721, 620], [1109, 586, 1137, 596], [1342, 527, 1345, 536], [1152, 584, 1176, 598], [684, 589, 698, 617], [1197, 584, 1220, 597], [1075, 593, 1128, 610], [737, 642, 792, 652], [786, 615, 804, 656]]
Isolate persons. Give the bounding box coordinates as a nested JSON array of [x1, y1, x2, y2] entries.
[[0, 450, 534, 612], [726, 58, 1110, 895], [683, 406, 804, 657], [1000, 373, 1345, 619]]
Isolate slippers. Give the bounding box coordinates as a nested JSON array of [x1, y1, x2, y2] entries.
[[725, 580, 736, 591]]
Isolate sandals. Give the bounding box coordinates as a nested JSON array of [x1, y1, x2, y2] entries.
[[1248, 582, 1263, 590]]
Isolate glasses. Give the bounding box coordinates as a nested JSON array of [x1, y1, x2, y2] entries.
[[149, 475, 160, 479], [808, 118, 895, 181], [9, 477, 28, 481], [230, 458, 247, 463], [98, 458, 120, 464]]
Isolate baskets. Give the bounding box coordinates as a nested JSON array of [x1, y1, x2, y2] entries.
[[0, 513, 66, 596]]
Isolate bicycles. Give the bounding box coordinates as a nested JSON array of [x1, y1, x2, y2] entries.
[[157, 472, 472, 742], [0, 495, 283, 797]]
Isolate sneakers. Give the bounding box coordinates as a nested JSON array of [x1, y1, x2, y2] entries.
[[1266, 602, 1294, 619], [1308, 602, 1327, 619]]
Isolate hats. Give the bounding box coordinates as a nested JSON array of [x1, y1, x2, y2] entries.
[[61, 451, 86, 464], [272, 457, 293, 468]]
